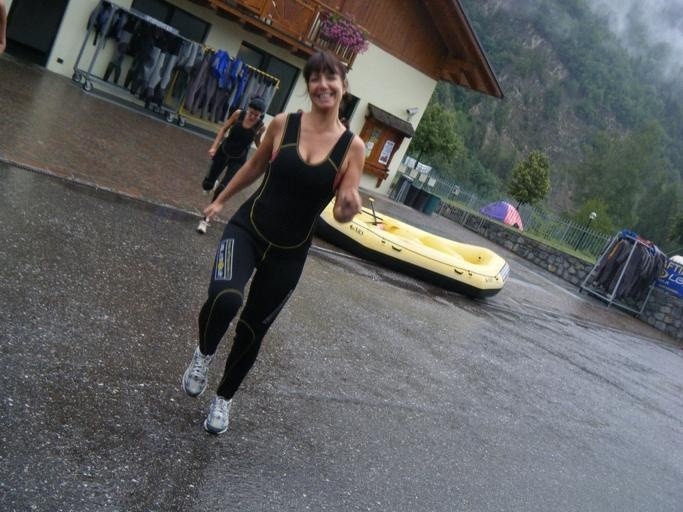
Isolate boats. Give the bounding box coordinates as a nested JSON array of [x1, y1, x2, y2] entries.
[[315, 192, 511, 298]]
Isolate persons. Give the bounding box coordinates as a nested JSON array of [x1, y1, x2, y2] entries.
[[178, 53, 368, 439], [194, 94, 265, 234]]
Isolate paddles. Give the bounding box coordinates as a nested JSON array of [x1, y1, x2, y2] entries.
[[361, 198, 401, 234]]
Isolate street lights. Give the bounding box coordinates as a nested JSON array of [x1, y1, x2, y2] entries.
[[573, 208, 598, 253]]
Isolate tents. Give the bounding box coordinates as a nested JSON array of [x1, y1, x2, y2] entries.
[[481, 198, 524, 232]]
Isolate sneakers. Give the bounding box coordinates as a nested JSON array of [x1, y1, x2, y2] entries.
[[203, 180, 218, 195], [182, 345, 218, 397], [197, 220, 207, 233], [204, 396, 232, 435]]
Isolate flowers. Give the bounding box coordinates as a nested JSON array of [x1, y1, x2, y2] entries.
[[318, 5, 375, 56]]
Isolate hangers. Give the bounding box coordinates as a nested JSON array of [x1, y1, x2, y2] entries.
[[243, 64, 277, 92]]
